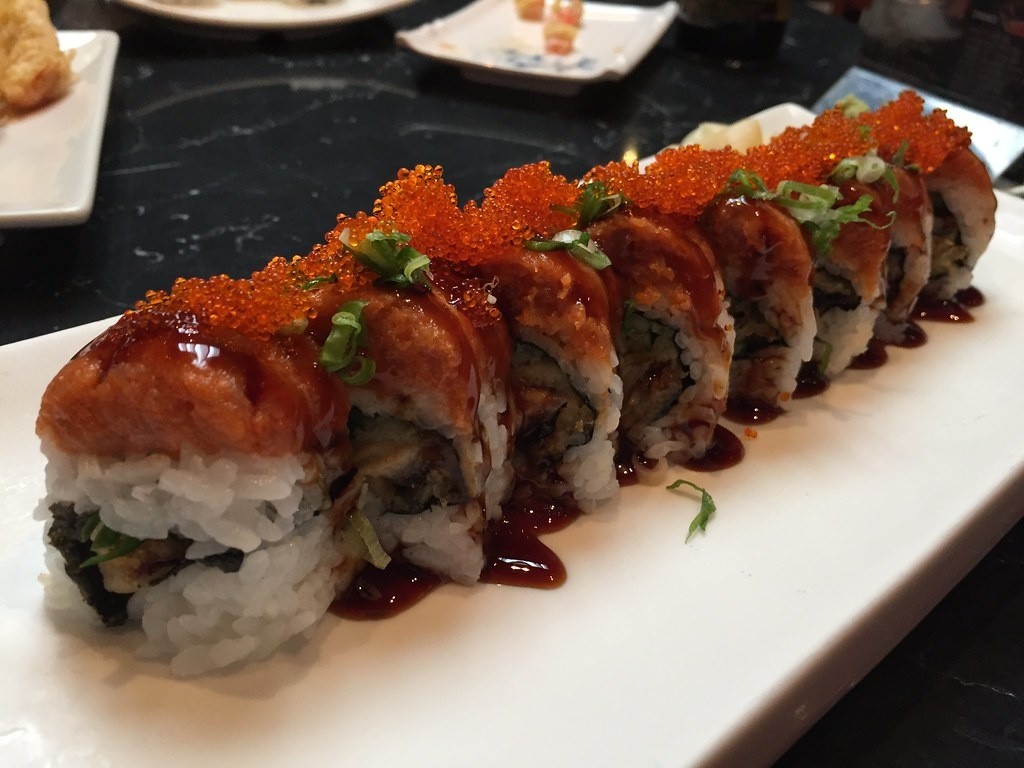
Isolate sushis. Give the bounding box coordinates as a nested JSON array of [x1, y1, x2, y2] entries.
[[31, 88, 999, 676]]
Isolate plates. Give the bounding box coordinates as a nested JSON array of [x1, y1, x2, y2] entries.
[[0, 100, 1024, 768], [0, 29, 122, 229], [393, 0, 680, 95], [116, 0, 419, 33]]
